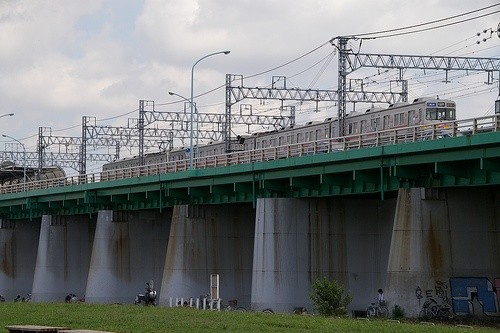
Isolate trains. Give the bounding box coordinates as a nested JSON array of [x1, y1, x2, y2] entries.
[[102, 97, 458, 183]]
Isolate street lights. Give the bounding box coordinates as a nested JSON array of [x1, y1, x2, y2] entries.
[[168, 91, 199, 169], [2, 134, 26, 192], [190, 50, 231, 169]]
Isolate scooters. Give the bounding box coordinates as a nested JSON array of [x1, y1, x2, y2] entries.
[[135, 279, 158, 306]]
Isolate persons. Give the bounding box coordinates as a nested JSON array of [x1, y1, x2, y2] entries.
[[14, 293, 31, 302], [378, 289, 384, 302], [65, 293, 78, 302], [178, 295, 211, 309]]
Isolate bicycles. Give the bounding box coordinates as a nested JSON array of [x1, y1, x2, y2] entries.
[[367, 297, 389, 319], [419, 301, 453, 322]]
